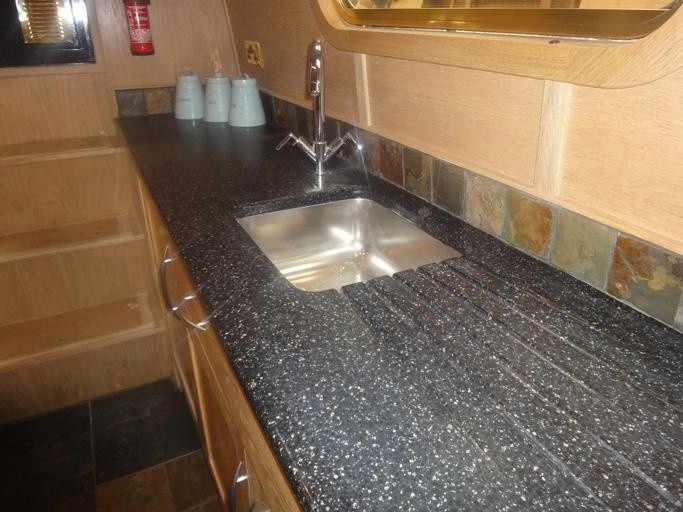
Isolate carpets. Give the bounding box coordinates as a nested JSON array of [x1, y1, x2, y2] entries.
[[96, 448, 227, 512]]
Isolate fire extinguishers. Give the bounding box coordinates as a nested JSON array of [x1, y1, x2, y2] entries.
[[123, 0, 154, 55]]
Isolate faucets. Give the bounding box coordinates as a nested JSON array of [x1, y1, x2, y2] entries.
[[307, 39, 328, 173]]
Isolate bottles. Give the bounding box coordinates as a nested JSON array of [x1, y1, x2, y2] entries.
[[172, 70, 267, 127]]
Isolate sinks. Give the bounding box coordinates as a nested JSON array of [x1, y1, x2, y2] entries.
[[232, 185, 474, 293]]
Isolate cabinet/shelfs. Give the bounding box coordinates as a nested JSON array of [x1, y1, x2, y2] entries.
[[137, 176, 302, 512]]
